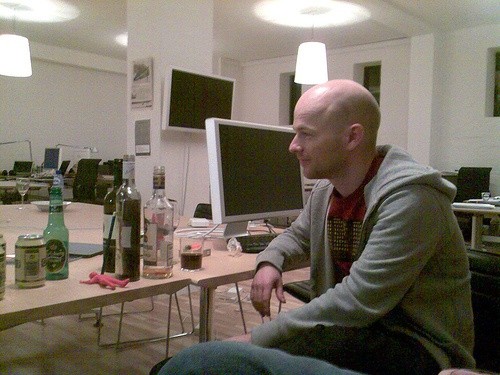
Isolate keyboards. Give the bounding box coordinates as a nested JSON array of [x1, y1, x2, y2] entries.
[[226, 232, 281, 253]]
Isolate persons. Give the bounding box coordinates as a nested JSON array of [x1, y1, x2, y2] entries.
[[79, 272, 129, 290], [157, 80, 476, 375]]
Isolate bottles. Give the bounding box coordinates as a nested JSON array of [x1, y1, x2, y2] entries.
[[142, 167, 173, 280], [54, 170, 64, 201], [44, 186, 69, 280], [115, 155, 142, 282], [102, 158, 123, 273]]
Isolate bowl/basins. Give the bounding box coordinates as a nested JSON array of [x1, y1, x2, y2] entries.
[[31, 201, 71, 212]]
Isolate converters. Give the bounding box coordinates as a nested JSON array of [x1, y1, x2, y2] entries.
[[189, 218, 209, 227]]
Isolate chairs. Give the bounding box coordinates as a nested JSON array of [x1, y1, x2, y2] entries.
[[450, 167, 492, 238], [74, 159, 99, 201]]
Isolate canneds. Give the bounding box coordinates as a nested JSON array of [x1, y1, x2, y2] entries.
[[14, 234, 46, 288], [0, 234, 7, 301]]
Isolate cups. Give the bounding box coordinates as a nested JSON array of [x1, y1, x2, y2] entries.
[[180, 233, 204, 272]]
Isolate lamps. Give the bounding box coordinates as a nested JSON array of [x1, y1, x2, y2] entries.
[[294, 9, 328, 84], [0, 8, 32, 77], [56, 144, 98, 153]]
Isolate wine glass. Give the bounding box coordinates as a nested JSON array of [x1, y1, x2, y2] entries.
[[155, 199, 180, 265], [16, 178, 30, 210]]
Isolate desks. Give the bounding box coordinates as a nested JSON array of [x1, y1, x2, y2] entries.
[[0, 202, 260, 344], [450, 197, 500, 250]]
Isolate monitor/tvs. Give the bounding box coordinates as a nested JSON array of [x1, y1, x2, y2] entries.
[[204, 117, 306, 237], [161, 67, 235, 133]]
